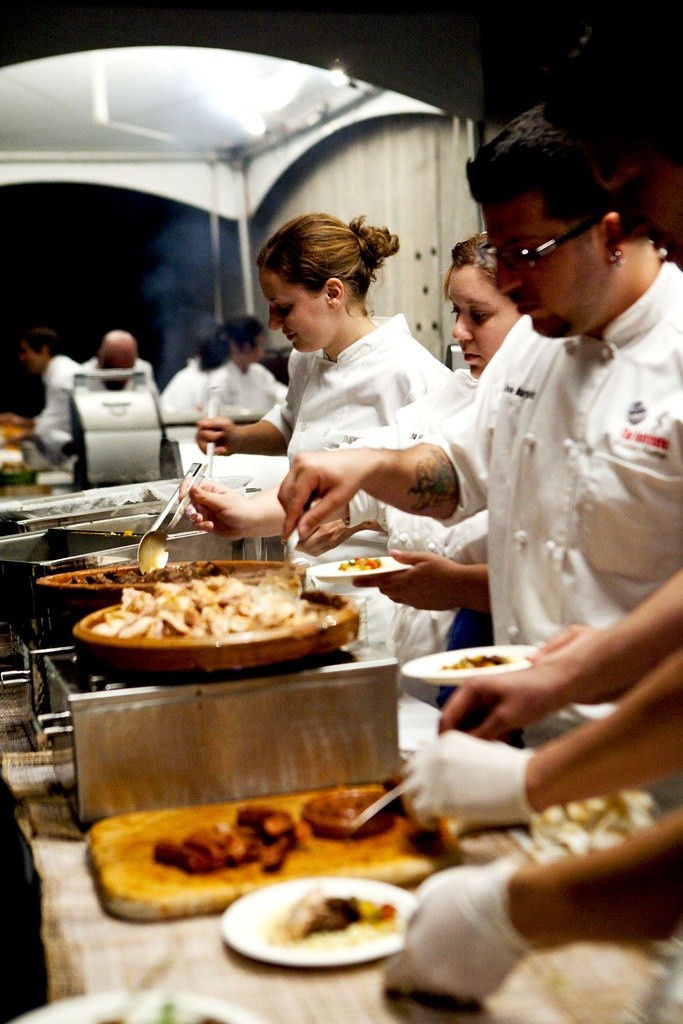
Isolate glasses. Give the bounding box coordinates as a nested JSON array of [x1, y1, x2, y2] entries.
[[478, 212, 604, 273]]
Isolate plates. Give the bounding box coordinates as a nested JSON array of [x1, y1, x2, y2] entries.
[[400, 645, 540, 685], [7, 988, 265, 1024], [219, 876, 419, 967], [306, 556, 413, 583]]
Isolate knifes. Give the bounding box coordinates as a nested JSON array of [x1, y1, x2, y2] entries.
[[352, 781, 410, 836]]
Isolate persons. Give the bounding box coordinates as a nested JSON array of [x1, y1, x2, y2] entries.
[[279, 96, 681, 746], [195, 211, 455, 663], [189, 234, 521, 746], [394, 566, 683, 835], [381, 810, 682, 1008], [35, 329, 159, 474], [15, 324, 82, 437], [159, 315, 288, 477]]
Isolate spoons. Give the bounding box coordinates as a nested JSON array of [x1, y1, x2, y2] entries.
[[137, 463, 210, 574]]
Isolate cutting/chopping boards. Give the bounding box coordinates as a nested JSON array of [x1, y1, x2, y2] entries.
[[84, 783, 463, 921]]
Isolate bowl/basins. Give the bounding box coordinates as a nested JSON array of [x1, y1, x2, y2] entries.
[[74, 591, 360, 672], [35, 560, 308, 606]]
